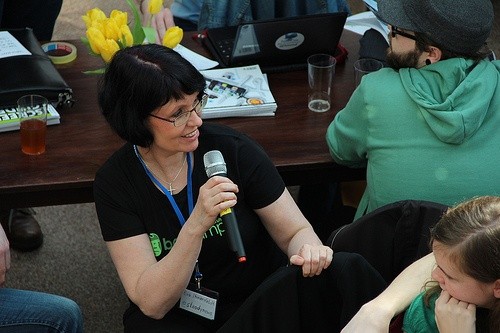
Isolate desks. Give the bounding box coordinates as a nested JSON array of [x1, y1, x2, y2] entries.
[[0, 10, 367, 208]]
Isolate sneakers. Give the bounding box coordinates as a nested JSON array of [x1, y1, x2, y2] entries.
[[0, 207, 43, 250]]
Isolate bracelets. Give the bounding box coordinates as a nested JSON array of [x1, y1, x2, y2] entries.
[[140, 0, 144, 15]]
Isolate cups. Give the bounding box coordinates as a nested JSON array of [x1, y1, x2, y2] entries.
[[16, 94, 47, 156], [353, 58, 383, 89], [307, 54, 336, 112]]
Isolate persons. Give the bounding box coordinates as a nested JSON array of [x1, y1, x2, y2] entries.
[[0, 0, 63, 252], [94, 44, 334, 333], [0, 223, 81, 333], [136, 0, 353, 45], [326, 0, 500, 220], [339, 196, 499, 333]]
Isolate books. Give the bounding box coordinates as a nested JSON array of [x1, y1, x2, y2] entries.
[[0, 104, 60, 132], [197, 64, 278, 119]]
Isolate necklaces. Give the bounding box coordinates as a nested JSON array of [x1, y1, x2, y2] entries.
[[141, 153, 187, 196]]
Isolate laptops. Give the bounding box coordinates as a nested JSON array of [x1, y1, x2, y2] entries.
[[205, 11, 347, 68]]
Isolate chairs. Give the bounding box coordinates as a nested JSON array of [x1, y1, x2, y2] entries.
[[233, 198, 457, 333]]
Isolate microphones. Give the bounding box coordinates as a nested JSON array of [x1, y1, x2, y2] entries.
[[204, 150, 246, 263]]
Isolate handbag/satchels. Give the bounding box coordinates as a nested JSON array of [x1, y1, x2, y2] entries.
[[0, 26, 76, 109]]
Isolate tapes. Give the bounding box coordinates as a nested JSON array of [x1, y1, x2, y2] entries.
[[41, 41, 77, 65]]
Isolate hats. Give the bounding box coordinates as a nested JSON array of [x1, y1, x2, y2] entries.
[[377, 0, 494, 55]]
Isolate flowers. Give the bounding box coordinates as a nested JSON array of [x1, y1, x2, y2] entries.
[[80, 0, 183, 74]]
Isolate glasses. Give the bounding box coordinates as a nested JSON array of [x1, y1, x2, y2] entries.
[[147, 92, 209, 126], [391, 25, 429, 46]]
